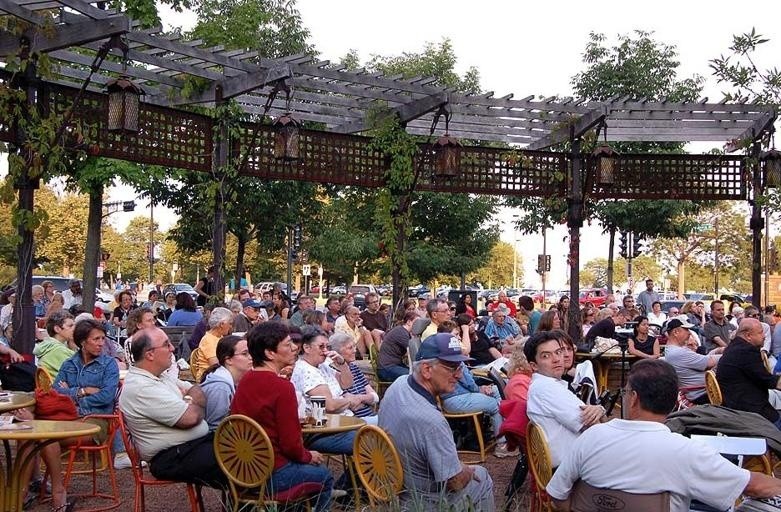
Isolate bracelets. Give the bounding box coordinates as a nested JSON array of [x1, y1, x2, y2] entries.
[[78, 386, 86, 398]]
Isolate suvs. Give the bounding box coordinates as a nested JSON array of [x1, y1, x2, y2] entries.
[[348, 285, 382, 309]]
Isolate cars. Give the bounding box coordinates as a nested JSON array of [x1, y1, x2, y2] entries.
[[579, 289, 607, 309], [309, 286, 334, 293], [373, 284, 472, 302], [162, 283, 199, 302], [477, 290, 502, 301], [502, 288, 571, 312], [699, 293, 754, 316], [255, 282, 297, 302], [653, 291, 703, 319], [94, 288, 112, 303], [27, 275, 84, 295], [331, 286, 348, 294]]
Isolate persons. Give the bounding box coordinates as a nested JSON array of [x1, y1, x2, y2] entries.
[[415, 320, 520, 463], [47, 294, 65, 315], [403, 298, 417, 314], [229, 270, 249, 290], [339, 296, 355, 315], [288, 296, 315, 329], [228, 300, 243, 320], [1, 288, 17, 330], [229, 320, 336, 512], [447, 301, 456, 316], [519, 296, 543, 337], [597, 308, 613, 319], [376, 313, 422, 384], [335, 305, 367, 345], [273, 291, 293, 319], [142, 291, 159, 309], [668, 307, 679, 318], [62, 278, 84, 309], [33, 309, 78, 384], [308, 310, 330, 331], [421, 299, 451, 343], [703, 300, 736, 354], [557, 295, 570, 332], [458, 313, 504, 367], [537, 309, 560, 331], [680, 301, 705, 337], [455, 293, 478, 321], [235, 298, 267, 331], [525, 331, 609, 475], [263, 292, 272, 301], [545, 358, 781, 512], [377, 332, 495, 512], [262, 300, 282, 321], [196, 306, 235, 383], [32, 284, 46, 316], [484, 310, 518, 345], [744, 306, 771, 367], [693, 301, 709, 322], [664, 316, 717, 405], [714, 317, 781, 430], [584, 301, 595, 309], [630, 309, 639, 320], [772, 322, 780, 358], [167, 292, 204, 326], [195, 265, 216, 306], [290, 324, 375, 507], [109, 290, 140, 326], [415, 294, 428, 318], [359, 292, 388, 332], [197, 335, 254, 434], [580, 307, 595, 338], [119, 327, 236, 512], [607, 304, 618, 316], [1, 320, 26, 364], [605, 294, 615, 306], [238, 289, 251, 304], [158, 291, 176, 322], [29, 319, 120, 511], [626, 316, 660, 368], [553, 328, 598, 404], [494, 302, 523, 337], [379, 304, 389, 316], [43, 280, 56, 308], [188, 303, 216, 353], [584, 309, 632, 350], [491, 292, 517, 318], [325, 297, 341, 323], [436, 320, 521, 458], [635, 278, 660, 316], [725, 302, 740, 321], [124, 307, 176, 366], [479, 300, 494, 316], [646, 302, 666, 327], [620, 295, 639, 313]]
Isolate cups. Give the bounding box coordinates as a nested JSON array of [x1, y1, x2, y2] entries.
[[311, 396, 326, 425], [363, 354, 368, 365]]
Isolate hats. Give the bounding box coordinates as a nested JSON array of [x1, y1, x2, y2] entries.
[[665, 316, 696, 330], [418, 293, 428, 301], [242, 299, 265, 308], [494, 303, 511, 316], [413, 332, 476, 364]]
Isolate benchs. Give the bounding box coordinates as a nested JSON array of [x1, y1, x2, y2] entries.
[[156, 326, 197, 359]]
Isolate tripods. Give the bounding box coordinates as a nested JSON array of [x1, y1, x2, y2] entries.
[[591, 321, 637, 424]]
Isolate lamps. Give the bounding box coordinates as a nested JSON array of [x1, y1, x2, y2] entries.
[[758, 149, 781, 188], [433, 136, 463, 178], [105, 77, 146, 136], [591, 146, 620, 185], [272, 115, 301, 160]]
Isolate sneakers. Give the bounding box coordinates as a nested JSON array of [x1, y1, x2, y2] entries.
[[494, 442, 521, 459], [113, 457, 148, 470]]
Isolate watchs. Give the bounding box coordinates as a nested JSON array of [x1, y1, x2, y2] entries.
[[334, 359, 348, 369], [596, 403, 607, 415], [183, 394, 195, 404]]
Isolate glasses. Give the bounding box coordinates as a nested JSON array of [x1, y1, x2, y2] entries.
[[625, 300, 635, 303], [433, 308, 450, 313], [624, 316, 630, 322], [671, 311, 680, 314], [147, 339, 169, 351], [310, 345, 332, 349], [449, 307, 458, 311], [587, 313, 595, 316], [233, 350, 250, 356], [748, 312, 761, 318]]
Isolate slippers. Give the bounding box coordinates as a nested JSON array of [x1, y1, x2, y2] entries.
[[22, 495, 37, 509], [49, 495, 77, 511]]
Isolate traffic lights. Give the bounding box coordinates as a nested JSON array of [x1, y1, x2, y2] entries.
[[294, 223, 302, 252], [633, 229, 643, 258], [123, 200, 137, 212], [538, 255, 551, 272], [620, 229, 629, 259], [146, 242, 152, 260], [101, 253, 110, 261]]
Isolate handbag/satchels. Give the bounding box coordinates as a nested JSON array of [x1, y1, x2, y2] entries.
[[33, 368, 79, 422]]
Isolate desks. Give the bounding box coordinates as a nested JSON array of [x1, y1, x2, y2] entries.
[[0, 392, 36, 413], [297, 410, 366, 511], [0, 419, 102, 512], [472, 367, 509, 380], [574, 352, 637, 408]]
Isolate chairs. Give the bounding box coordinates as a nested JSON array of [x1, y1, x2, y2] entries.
[[212, 413, 323, 512], [115, 408, 206, 512], [189, 346, 199, 381], [369, 343, 392, 396], [526, 421, 554, 511], [704, 371, 781, 477], [435, 393, 499, 464], [34, 367, 54, 397], [352, 422, 404, 512], [37, 377, 126, 512], [319, 452, 361, 511]]
